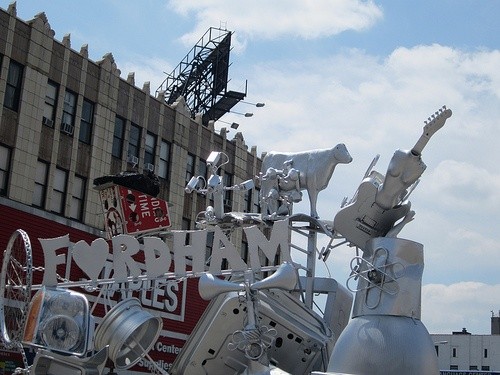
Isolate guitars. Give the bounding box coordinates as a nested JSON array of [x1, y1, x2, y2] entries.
[[331, 104, 452, 255]]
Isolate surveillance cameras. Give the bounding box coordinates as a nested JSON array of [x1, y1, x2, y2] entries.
[[184, 175, 199, 194], [239, 179, 254, 192], [205, 150, 221, 169], [208, 174, 220, 193]]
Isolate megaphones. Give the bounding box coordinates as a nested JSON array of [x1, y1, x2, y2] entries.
[[249, 260, 299, 292], [198, 273, 250, 301]]
